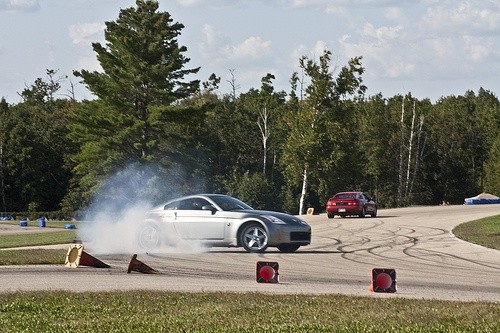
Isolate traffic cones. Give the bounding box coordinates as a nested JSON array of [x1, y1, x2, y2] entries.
[[127, 254, 160, 274], [368, 268, 397, 293], [306, 208, 319, 215], [64, 244, 111, 269], [255, 261, 279, 284]]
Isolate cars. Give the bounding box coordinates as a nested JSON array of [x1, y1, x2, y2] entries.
[[141, 194, 312, 253], [326, 192, 377, 218]]
